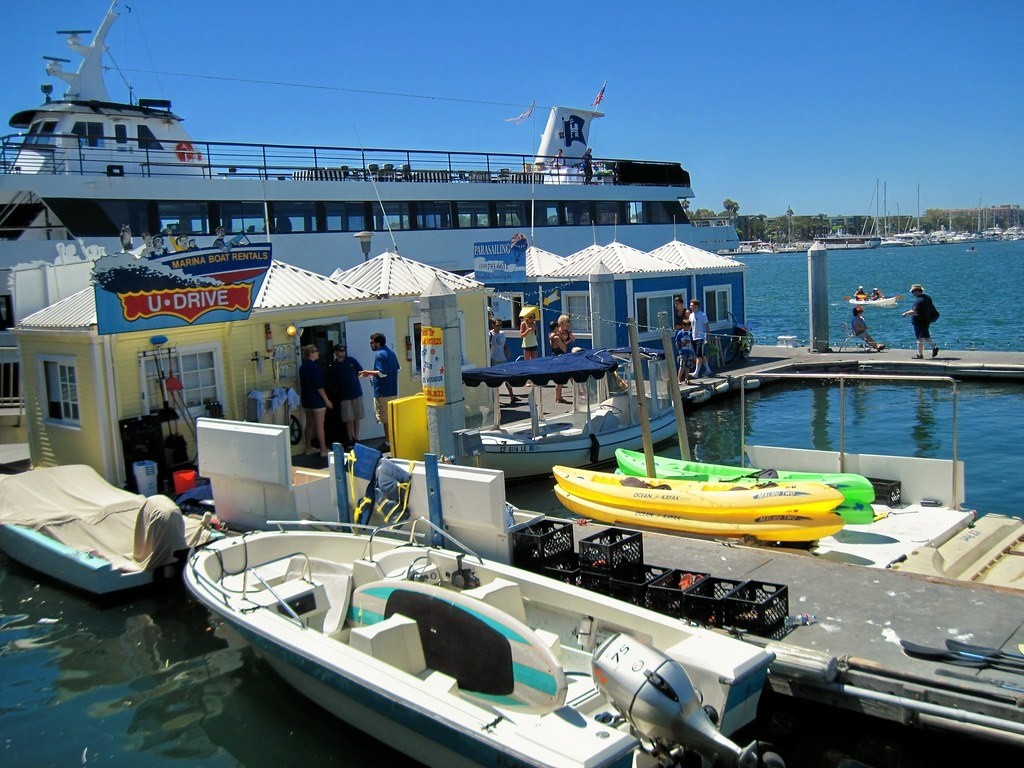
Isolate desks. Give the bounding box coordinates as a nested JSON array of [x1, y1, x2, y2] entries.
[[530, 169, 613, 185]]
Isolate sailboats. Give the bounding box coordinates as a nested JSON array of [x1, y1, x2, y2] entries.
[[717, 178, 1024, 257]]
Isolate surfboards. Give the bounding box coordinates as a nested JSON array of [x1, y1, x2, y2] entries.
[[346, 578, 568, 712]]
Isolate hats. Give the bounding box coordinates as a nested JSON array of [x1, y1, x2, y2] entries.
[[859, 286, 863, 288], [333, 343, 345, 352], [488, 307, 492, 311], [549, 321, 558, 331], [853, 307, 863, 314], [873, 288, 878, 290], [909, 285, 921, 291]]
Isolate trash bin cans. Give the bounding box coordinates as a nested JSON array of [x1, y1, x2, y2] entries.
[[134, 461, 159, 496]]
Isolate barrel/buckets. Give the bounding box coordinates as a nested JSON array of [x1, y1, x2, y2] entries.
[[133, 461, 158, 497], [173, 469, 196, 496]]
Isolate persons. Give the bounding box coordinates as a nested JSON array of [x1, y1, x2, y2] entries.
[[520, 312, 538, 387], [674, 297, 693, 385], [870, 287, 879, 301], [548, 315, 574, 402], [853, 286, 869, 301], [551, 149, 564, 167], [902, 284, 940, 359], [581, 147, 593, 185], [487, 306, 495, 349], [276, 210, 292, 232], [488, 318, 522, 403], [299, 344, 333, 456], [687, 299, 711, 378], [851, 307, 885, 351], [327, 343, 365, 449], [358, 332, 400, 453]]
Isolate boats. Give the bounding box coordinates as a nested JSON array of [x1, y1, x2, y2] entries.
[[451, 345, 678, 487], [552, 483, 845, 544], [552, 464, 848, 520], [614, 447, 877, 525], [182, 515, 787, 767], [0, 464, 228, 601], [0, 0, 743, 408], [849, 296, 896, 307]]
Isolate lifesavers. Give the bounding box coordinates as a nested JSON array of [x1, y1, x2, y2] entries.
[[177, 142, 194, 161]]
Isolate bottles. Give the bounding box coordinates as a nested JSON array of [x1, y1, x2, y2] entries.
[[920, 498, 943, 506], [783, 613, 816, 626], [205, 401, 222, 417]]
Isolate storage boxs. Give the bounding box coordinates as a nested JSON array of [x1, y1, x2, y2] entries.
[[581, 569, 611, 596], [609, 563, 674, 608], [649, 569, 712, 618], [195, 417, 338, 532], [579, 528, 643, 576], [512, 520, 574, 568], [326, 452, 545, 567], [683, 577, 745, 628], [541, 552, 581, 586], [724, 580, 789, 637]]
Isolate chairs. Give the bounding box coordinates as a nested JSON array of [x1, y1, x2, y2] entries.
[[839, 322, 865, 352], [369, 164, 510, 183]]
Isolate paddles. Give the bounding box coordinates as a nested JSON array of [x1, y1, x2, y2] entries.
[[843, 295, 856, 300], [880, 295, 904, 300]]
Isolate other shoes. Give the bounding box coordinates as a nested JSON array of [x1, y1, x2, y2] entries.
[[305, 448, 321, 455], [684, 380, 690, 385], [688, 373, 698, 378], [343, 440, 355, 448], [377, 442, 390, 452], [932, 347, 938, 357], [677, 381, 682, 385], [703, 374, 713, 379], [321, 449, 331, 456], [510, 397, 522, 403], [877, 344, 885, 352], [556, 399, 567, 403], [911, 354, 923, 359]]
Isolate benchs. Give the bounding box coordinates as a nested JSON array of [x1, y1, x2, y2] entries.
[[220, 554, 304, 597]]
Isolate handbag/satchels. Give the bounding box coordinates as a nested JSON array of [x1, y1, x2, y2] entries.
[[931, 306, 939, 322]]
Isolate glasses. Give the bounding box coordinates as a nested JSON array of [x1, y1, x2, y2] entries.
[[370, 342, 377, 345], [311, 350, 319, 353], [336, 349, 345, 352], [566, 320, 570, 323]]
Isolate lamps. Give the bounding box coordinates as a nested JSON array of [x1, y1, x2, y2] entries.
[[287, 325, 297, 337], [40, 83, 53, 102]]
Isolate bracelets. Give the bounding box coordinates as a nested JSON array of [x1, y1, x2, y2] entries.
[[569, 335, 573, 337]]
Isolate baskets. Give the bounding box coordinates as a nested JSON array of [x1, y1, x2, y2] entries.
[[732, 326, 746, 338]]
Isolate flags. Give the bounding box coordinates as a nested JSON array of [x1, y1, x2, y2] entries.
[[504, 101, 534, 127], [588, 83, 605, 106]]
